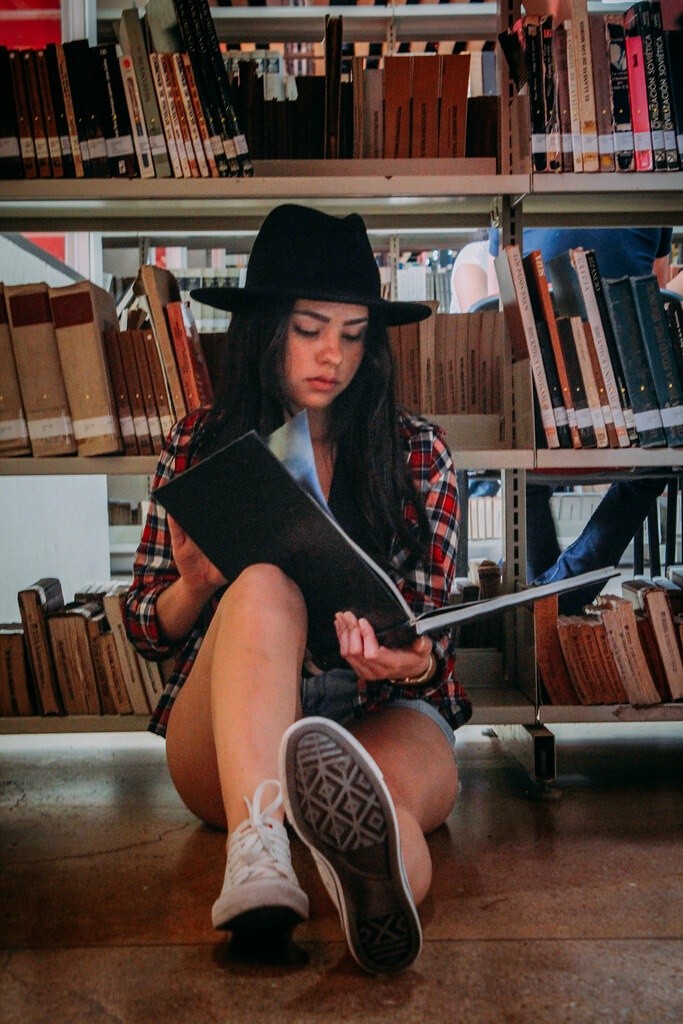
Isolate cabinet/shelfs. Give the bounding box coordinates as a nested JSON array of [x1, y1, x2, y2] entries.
[[0, 0, 683, 807]]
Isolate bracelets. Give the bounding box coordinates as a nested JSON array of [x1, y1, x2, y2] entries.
[[389, 654, 433, 684]]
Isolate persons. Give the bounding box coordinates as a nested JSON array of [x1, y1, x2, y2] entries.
[[123, 204, 474, 977], [488, 226, 671, 614], [448, 238, 499, 315]]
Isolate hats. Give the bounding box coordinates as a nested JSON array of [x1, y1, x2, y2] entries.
[[189, 203, 432, 327]]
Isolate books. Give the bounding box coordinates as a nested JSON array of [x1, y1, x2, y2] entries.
[[151, 408, 621, 672], [0, 0, 683, 718]]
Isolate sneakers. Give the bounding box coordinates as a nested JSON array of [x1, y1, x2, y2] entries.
[[211, 779, 309, 929], [278, 715, 422, 975]]
